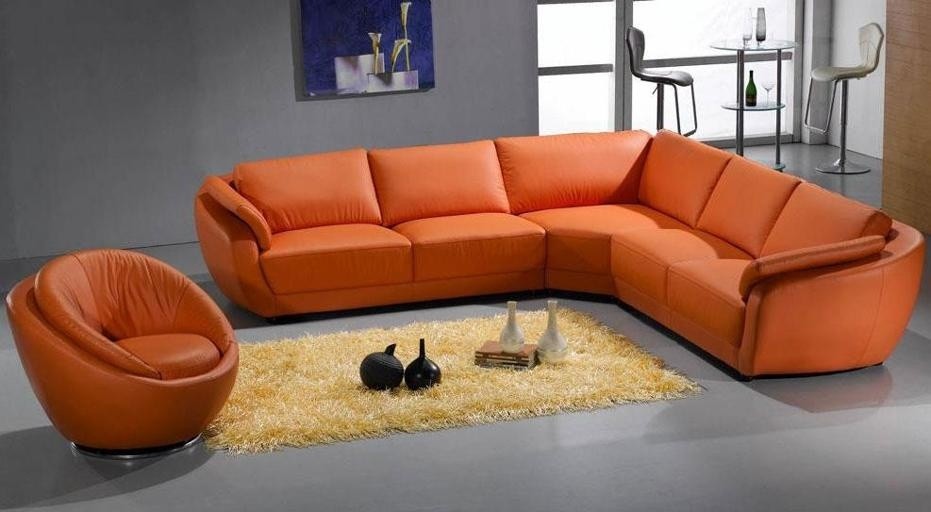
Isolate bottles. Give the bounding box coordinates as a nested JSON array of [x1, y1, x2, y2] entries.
[[500, 301, 524, 346], [538, 300, 568, 361], [746, 71, 758, 107], [743, 5, 767, 48]]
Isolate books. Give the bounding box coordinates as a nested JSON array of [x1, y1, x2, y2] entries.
[[474, 340, 539, 371]]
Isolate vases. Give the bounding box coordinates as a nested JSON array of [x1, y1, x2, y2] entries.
[[359, 336, 441, 393], [501, 295, 572, 358], [333, 53, 420, 95]]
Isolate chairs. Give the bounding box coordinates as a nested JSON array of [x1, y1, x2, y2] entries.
[[5, 248, 240, 461], [802, 21, 885, 175], [624, 23, 697, 137]]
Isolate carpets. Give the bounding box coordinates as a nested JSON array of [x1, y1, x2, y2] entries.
[[200, 305, 710, 457]]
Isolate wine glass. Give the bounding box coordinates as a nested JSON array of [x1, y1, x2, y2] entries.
[[761, 83, 775, 105]]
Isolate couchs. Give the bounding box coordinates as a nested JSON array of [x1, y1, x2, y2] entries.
[[194, 172, 926, 381]]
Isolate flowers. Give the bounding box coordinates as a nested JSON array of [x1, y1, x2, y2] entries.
[[366, 1, 413, 74]]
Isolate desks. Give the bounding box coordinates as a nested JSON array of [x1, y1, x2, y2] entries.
[[710, 40, 799, 170]]
